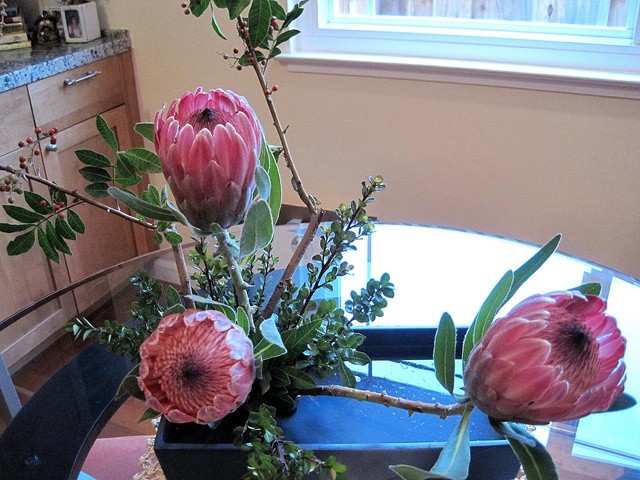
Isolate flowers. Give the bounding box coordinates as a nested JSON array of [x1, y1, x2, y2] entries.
[[0, 0, 640, 480]]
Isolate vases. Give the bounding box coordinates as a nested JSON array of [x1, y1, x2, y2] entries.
[[153, 323, 522, 479]]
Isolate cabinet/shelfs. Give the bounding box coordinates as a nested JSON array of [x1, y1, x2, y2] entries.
[[0, 83, 80, 421], [26, 49, 160, 321]]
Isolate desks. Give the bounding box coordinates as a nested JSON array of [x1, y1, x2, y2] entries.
[[0, 220, 640, 480]]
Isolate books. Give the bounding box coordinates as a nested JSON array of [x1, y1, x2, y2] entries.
[[0, 15, 32, 52]]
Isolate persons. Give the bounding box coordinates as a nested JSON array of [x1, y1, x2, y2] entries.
[[68, 17, 81, 37]]
